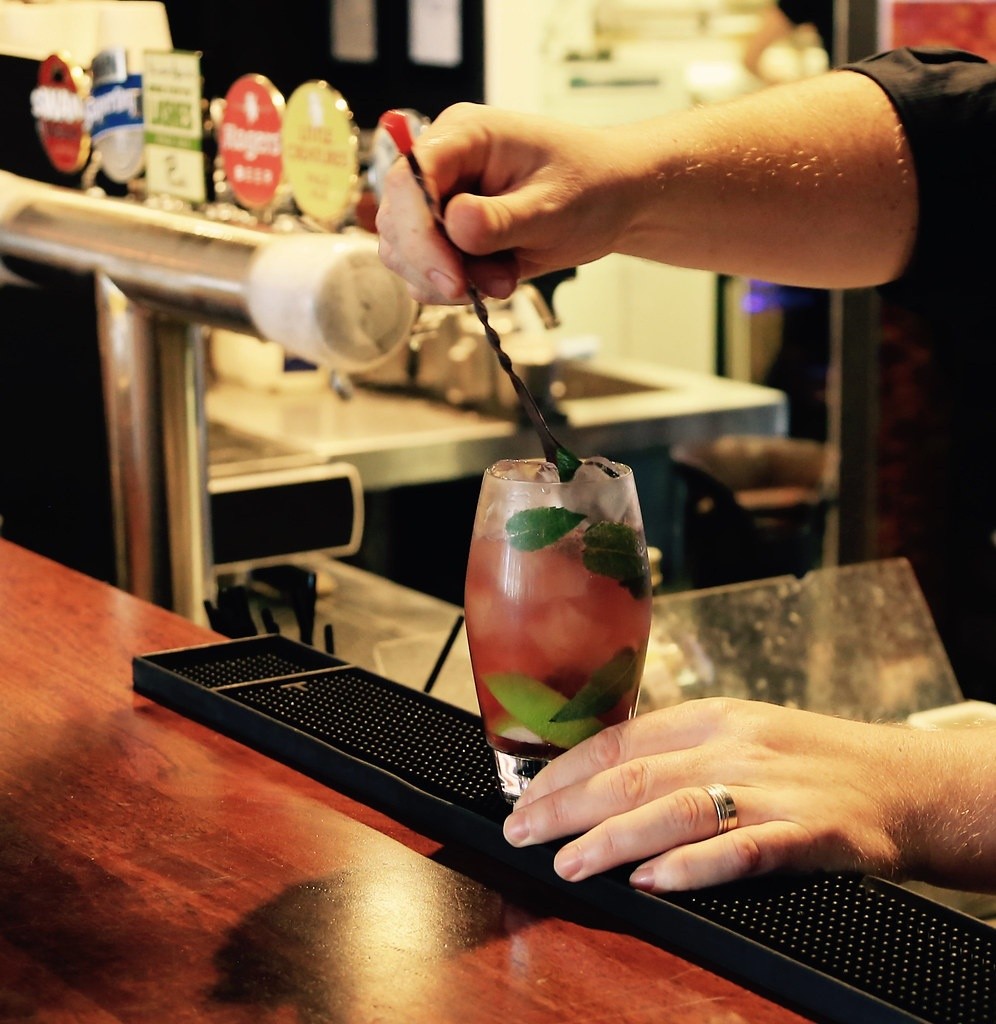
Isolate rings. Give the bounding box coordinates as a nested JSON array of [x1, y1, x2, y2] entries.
[[703, 783, 738, 835]]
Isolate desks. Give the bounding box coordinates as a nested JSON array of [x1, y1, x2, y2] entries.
[[0, 537, 821, 1024]]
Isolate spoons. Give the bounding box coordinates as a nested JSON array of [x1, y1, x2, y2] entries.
[[379, 109, 583, 483]]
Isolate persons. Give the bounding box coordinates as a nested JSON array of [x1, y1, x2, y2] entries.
[[375, 49, 995, 894]]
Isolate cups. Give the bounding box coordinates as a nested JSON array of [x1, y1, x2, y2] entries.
[[462, 456, 655, 803]]
